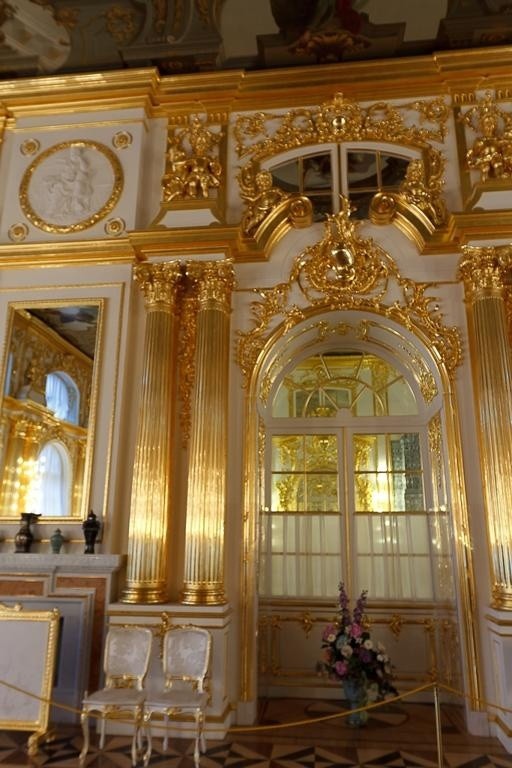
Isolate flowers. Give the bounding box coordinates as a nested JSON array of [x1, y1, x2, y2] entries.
[[310, 579, 405, 704]]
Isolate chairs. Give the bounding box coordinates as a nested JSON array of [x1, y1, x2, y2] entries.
[[78, 620, 215, 766]]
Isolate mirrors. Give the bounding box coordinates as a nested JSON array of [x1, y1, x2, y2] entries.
[[1, 296, 106, 525]]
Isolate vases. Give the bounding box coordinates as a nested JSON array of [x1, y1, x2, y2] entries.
[[342, 672, 370, 725]]
